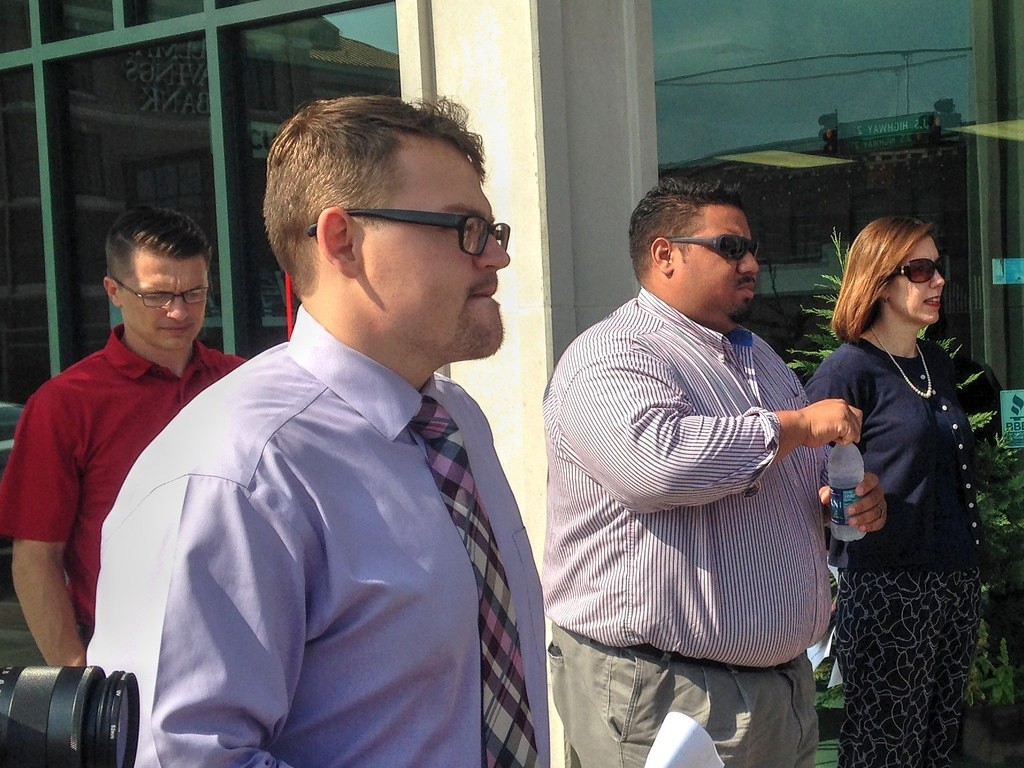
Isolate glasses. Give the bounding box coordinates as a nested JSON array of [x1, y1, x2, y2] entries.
[[889, 254, 951, 284], [673, 235, 762, 260], [113, 270, 215, 307], [307, 206, 513, 254]]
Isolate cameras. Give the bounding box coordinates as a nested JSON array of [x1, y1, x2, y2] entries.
[[0, 666, 140, 768]]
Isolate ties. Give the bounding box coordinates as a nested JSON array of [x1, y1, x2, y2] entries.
[[414, 393, 542, 768]]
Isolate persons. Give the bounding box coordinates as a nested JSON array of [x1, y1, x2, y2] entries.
[[87, 97, 549, 768], [805, 217, 982, 768], [0, 206, 245, 666], [543, 177, 887, 768]]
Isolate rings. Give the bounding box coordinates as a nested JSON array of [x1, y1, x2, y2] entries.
[[876, 505, 883, 516]]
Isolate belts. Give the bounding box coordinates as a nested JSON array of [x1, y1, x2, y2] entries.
[[627, 640, 799, 674]]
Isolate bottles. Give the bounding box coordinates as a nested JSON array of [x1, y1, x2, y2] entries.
[[828, 440, 866, 542]]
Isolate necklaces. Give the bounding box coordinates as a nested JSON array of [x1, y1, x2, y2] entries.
[[869, 326, 931, 400]]
